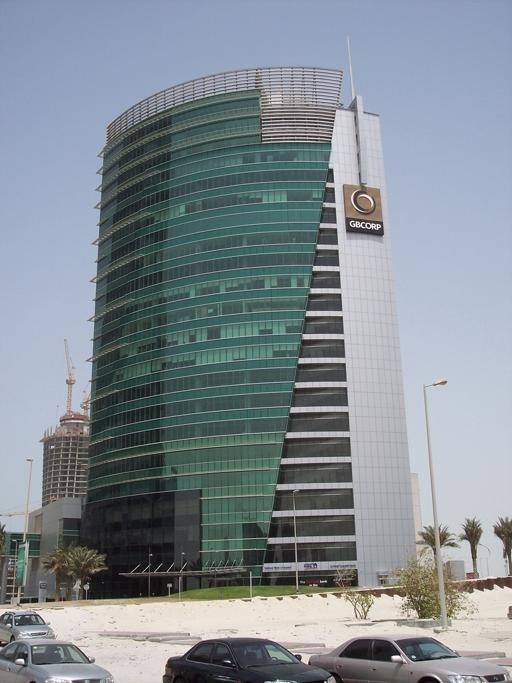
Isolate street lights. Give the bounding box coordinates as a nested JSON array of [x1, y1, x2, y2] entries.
[[23, 458, 34, 543], [293, 489, 300, 592], [422, 378, 449, 632]]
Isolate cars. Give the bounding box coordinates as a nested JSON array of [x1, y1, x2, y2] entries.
[[0, 610, 56, 646], [0, 638, 115, 683], [162, 637, 337, 683], [308, 633, 512, 683]]
